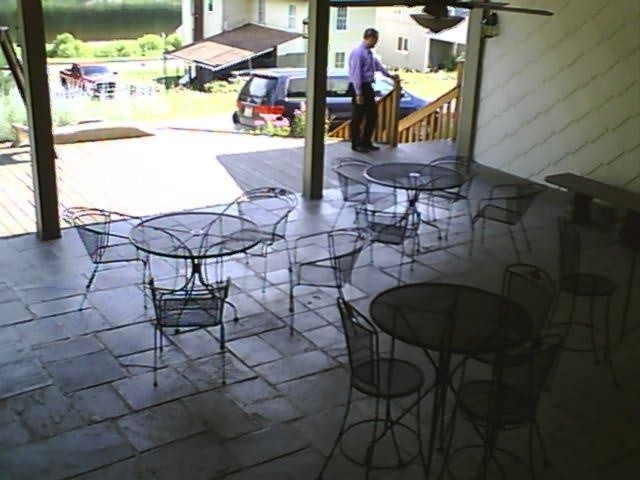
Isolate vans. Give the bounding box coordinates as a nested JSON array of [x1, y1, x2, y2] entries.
[[233, 66, 440, 138]]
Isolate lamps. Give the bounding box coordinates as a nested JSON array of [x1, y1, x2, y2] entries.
[[411, 5, 464, 31]]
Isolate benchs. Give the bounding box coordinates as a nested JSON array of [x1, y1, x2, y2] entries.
[[544, 170, 639, 245]]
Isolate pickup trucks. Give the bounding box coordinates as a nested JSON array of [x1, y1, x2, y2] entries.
[[60, 63, 118, 98]]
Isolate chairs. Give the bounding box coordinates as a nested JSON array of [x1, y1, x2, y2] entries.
[[196, 185, 298, 312], [468, 182, 548, 259], [148, 276, 232, 388], [541, 215, 620, 391], [437, 334, 551, 479], [329, 157, 411, 240], [287, 226, 378, 336], [620, 216, 639, 337], [313, 297, 431, 480], [409, 155, 482, 243], [61, 205, 156, 310], [350, 192, 418, 271], [464, 262, 561, 443]]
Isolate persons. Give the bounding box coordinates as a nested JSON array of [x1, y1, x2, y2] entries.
[[349, 28, 399, 153]]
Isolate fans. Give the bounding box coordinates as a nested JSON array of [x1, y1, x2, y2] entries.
[[321, 0, 553, 32]]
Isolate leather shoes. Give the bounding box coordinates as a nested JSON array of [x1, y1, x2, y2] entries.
[[352, 144, 371, 154], [366, 144, 381, 153]]
